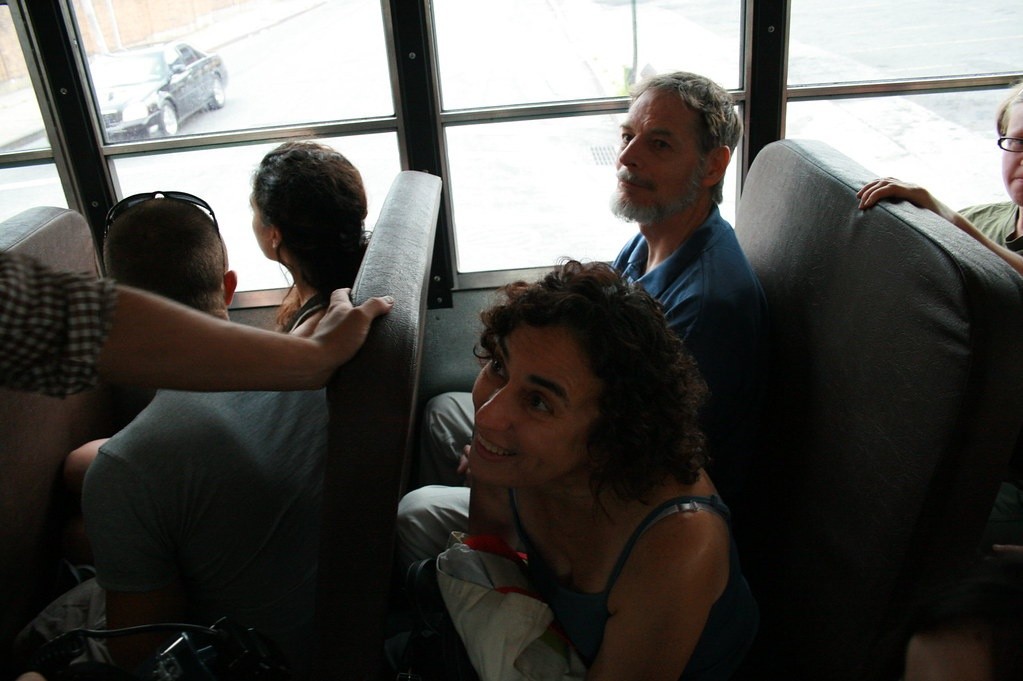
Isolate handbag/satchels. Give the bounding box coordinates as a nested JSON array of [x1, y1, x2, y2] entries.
[[29, 623, 298, 681], [392, 556, 480, 681]]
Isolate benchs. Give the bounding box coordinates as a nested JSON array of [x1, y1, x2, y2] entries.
[[731, 140, 1018, 681], [0, 208, 106, 644], [309, 171, 443, 681]]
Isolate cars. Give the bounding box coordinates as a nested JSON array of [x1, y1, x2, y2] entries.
[[90, 43, 228, 141]]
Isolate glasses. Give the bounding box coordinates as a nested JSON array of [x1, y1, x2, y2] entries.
[[103, 190, 219, 235], [998, 138, 1023, 153]]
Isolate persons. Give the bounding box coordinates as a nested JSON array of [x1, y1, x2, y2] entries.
[[903, 554, 1023, 681], [378, 260, 762, 681], [0, 142, 396, 681], [397, 76, 770, 585], [855, 81, 1023, 552]]
